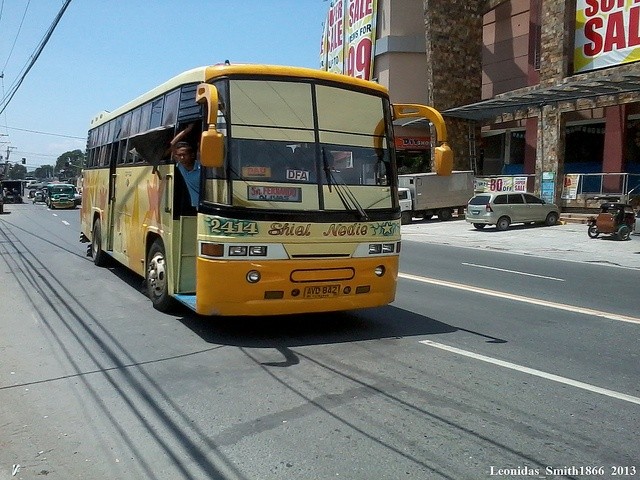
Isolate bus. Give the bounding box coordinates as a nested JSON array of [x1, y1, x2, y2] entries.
[[80, 60, 455, 317]]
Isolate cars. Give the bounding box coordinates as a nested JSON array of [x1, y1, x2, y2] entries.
[[73, 187, 81, 208], [25, 177, 46, 205], [42, 184, 74, 210], [464, 192, 560, 230]]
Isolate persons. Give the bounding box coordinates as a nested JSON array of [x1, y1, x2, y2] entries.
[[170, 123, 201, 212], [318, 151, 345, 185]]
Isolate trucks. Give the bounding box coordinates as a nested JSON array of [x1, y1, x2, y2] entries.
[[398, 171, 475, 221]]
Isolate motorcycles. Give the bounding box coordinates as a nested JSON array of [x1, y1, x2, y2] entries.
[[0, 188, 22, 204], [587, 196, 636, 241]]
